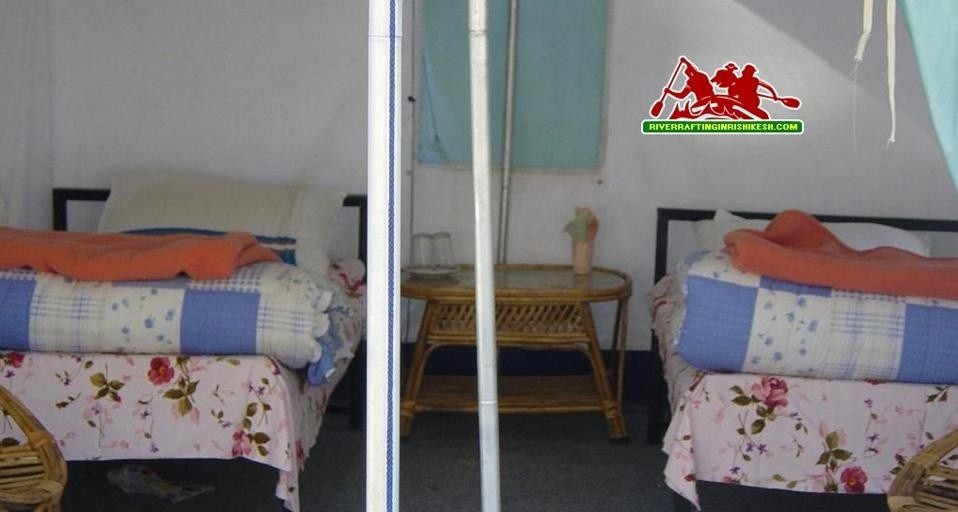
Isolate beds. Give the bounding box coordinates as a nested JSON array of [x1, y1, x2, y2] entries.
[[0, 186, 366, 512], [651, 203, 958, 512]]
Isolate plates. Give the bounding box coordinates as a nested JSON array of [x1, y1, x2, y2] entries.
[[407, 268, 460, 279]]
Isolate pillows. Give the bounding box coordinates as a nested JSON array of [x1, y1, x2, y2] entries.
[[95, 175, 349, 277], [691, 209, 932, 256]]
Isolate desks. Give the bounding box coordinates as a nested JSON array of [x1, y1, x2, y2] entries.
[[400, 262, 635, 445]]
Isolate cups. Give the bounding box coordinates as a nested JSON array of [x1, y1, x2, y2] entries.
[[409, 232, 455, 271], [572, 240, 594, 276]]
